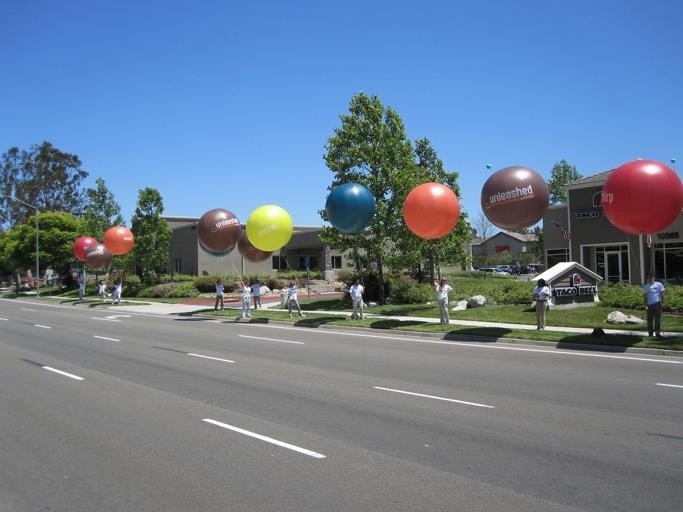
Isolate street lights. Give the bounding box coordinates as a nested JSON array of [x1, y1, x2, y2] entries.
[[2, 195, 39, 296]]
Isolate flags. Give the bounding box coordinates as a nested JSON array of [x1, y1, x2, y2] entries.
[[555, 223, 569, 240], [646, 235, 652, 248]]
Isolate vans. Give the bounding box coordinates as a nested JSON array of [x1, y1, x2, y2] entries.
[[479, 268, 511, 275]]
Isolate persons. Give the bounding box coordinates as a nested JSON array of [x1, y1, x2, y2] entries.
[[214, 280, 224, 311], [241, 281, 253, 318], [534, 278, 550, 332], [349, 278, 365, 320], [79, 280, 85, 300], [251, 280, 261, 309], [436, 278, 452, 324], [111, 280, 122, 304], [643, 272, 666, 336], [96, 280, 106, 302], [288, 278, 306, 318]]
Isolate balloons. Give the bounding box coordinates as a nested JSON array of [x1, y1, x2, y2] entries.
[[73, 236, 98, 261], [197, 205, 293, 262], [104, 225, 135, 255], [602, 160, 683, 235], [481, 166, 549, 230], [325, 182, 375, 235], [84, 243, 112, 270], [403, 182, 460, 240]]
